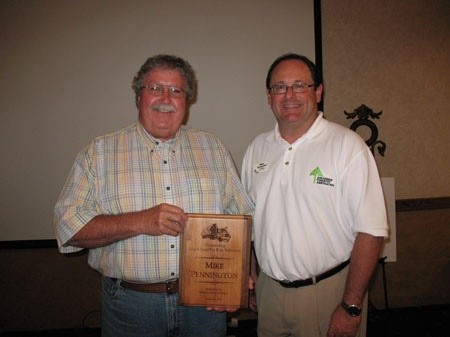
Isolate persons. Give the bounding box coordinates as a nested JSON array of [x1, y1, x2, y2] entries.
[[53, 54, 255, 337], [241, 53, 389, 337]]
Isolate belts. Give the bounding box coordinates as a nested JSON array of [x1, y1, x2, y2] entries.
[[272, 258, 351, 288], [111, 277, 178, 293]]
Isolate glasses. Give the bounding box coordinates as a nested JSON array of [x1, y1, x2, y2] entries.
[[269, 82, 314, 94], [139, 82, 187, 97]]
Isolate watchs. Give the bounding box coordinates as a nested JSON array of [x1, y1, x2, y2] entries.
[[340, 300, 362, 317]]
